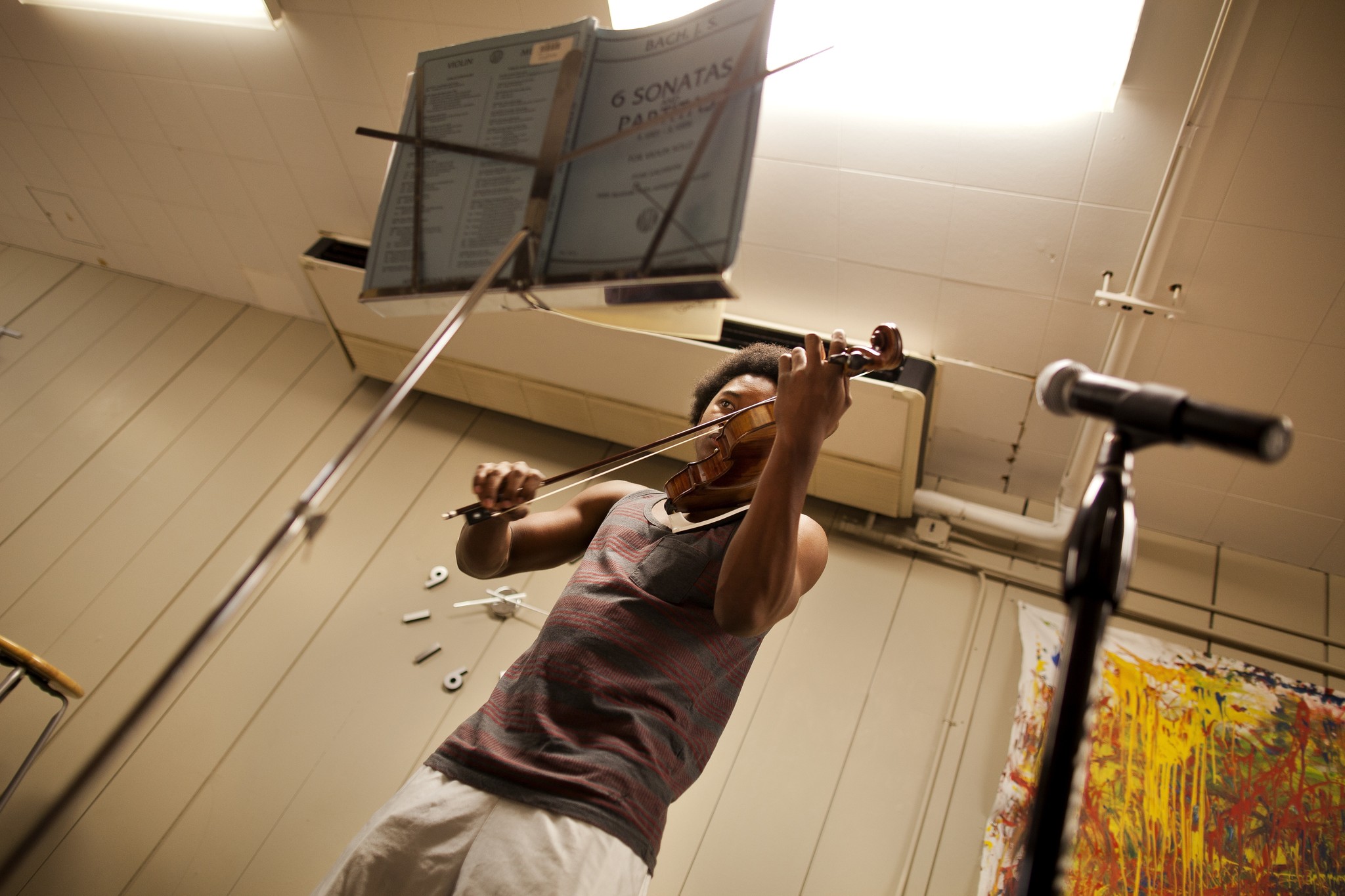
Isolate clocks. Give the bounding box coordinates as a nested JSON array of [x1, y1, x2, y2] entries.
[[399, 564, 552, 693]]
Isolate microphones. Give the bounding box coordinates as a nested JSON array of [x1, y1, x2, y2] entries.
[[1033, 359, 1297, 467]]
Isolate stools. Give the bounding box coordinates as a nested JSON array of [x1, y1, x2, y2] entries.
[[0, 636, 85, 814]]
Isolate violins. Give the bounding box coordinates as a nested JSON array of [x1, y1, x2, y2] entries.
[[663, 321, 905, 514]]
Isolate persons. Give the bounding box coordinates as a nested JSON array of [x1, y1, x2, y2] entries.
[[311, 329, 852, 896]]
